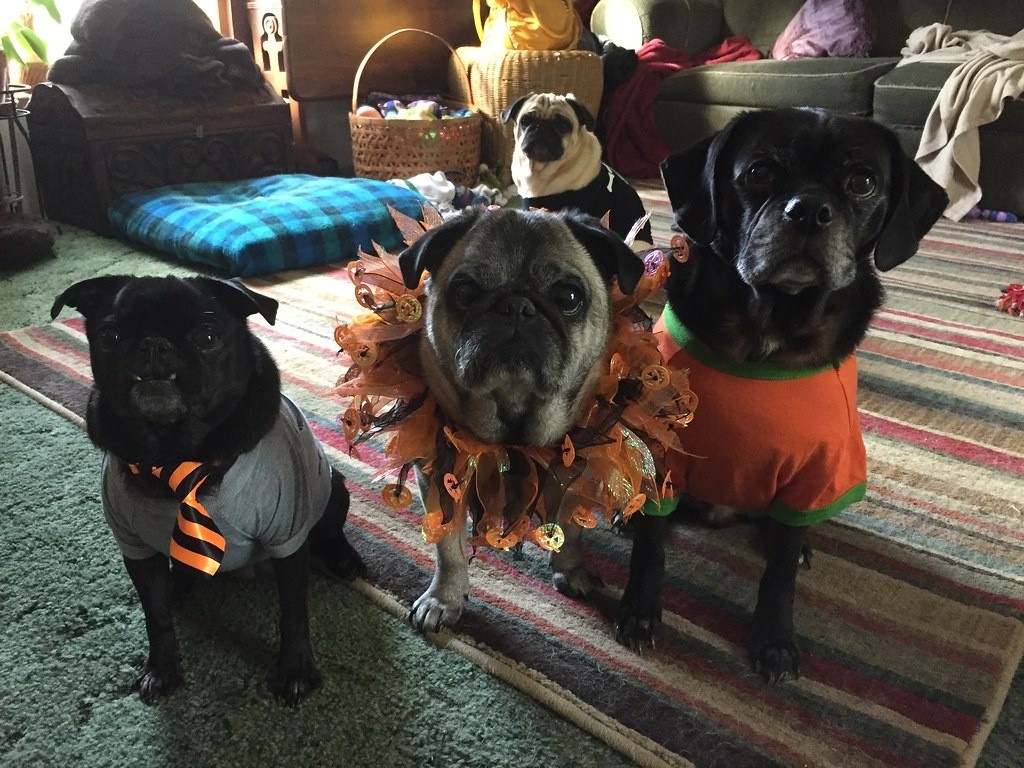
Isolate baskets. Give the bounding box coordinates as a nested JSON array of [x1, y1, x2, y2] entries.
[[349, 27, 482, 188]]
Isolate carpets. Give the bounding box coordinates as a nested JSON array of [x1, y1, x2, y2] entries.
[[5, 177, 1024, 768]]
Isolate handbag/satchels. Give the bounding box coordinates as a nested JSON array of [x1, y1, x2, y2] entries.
[[473, 0, 583, 52]]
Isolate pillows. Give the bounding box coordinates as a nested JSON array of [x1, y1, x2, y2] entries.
[[109, 172, 429, 281]]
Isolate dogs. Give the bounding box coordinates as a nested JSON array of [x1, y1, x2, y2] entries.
[[49, 272, 372, 712], [395, 203, 646, 638], [498, 90, 656, 254], [612, 102, 954, 690]]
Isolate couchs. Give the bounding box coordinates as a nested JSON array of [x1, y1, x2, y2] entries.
[[590, 0, 1024, 217]]
